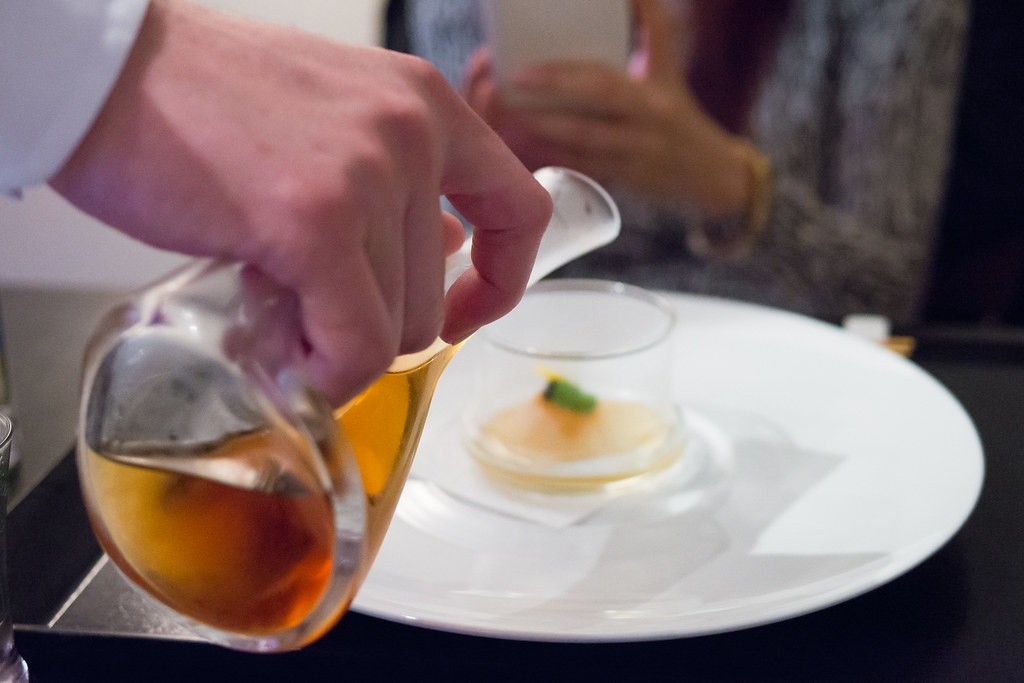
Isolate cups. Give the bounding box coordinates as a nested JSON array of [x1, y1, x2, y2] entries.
[[457, 278, 685, 493]]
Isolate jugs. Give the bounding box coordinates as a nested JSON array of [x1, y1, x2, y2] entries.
[[79, 162, 620, 654]]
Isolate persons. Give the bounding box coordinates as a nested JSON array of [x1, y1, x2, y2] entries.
[[459, 0, 970, 327], [1, 2, 557, 412]]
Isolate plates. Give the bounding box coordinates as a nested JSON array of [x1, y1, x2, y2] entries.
[[346, 289, 985, 641]]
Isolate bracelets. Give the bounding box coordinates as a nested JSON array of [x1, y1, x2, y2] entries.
[[683, 140, 768, 266]]
